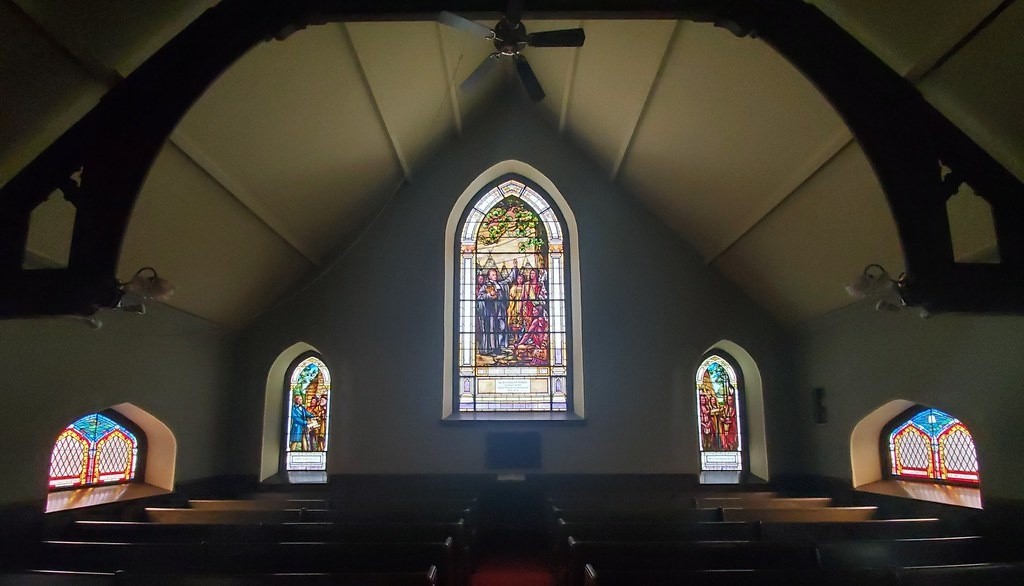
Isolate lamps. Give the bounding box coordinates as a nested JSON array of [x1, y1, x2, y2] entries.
[[845, 263, 912, 307], [110, 269, 176, 316]]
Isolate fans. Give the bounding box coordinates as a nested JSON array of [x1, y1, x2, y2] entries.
[[434, 7, 586, 105]]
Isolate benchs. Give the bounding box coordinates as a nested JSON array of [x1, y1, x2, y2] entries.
[[0, 471, 484, 586], [525, 471, 1024, 586]]
